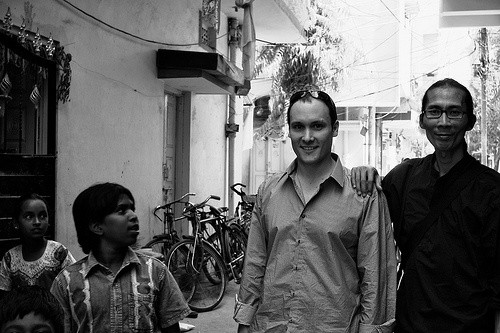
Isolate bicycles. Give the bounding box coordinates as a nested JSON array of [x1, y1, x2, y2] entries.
[[231, 182, 254, 227], [143, 193, 224, 303], [165, 195, 248, 314]]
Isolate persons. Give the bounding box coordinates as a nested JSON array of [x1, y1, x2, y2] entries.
[[233, 89, 397, 333], [350, 78, 500, 333], [0, 284, 65, 333], [49, 181, 192, 333], [0, 193, 80, 297]]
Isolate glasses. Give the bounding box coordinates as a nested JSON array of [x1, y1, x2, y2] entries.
[[425, 106, 466, 119]]
